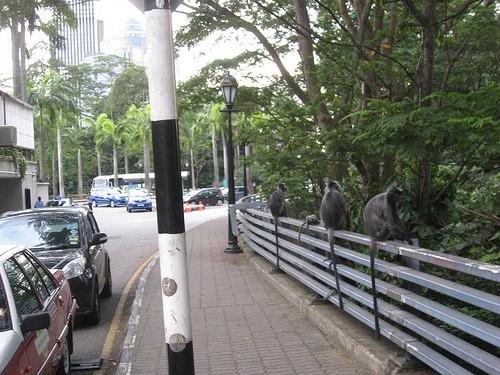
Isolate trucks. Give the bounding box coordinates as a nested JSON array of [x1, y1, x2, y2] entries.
[[90, 175, 123, 188]]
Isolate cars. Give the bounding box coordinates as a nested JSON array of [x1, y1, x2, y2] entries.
[[0, 244, 77, 375], [126, 188, 153, 212], [223, 186, 251, 201], [88, 190, 128, 207]]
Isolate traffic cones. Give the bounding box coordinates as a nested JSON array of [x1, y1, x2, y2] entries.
[[185, 202, 191, 211], [198, 201, 204, 210]]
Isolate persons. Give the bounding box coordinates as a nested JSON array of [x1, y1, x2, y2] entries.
[[87, 192, 93, 210], [34, 197, 44, 208]]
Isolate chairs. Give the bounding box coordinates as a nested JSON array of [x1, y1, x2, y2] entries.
[[48, 232, 71, 244]]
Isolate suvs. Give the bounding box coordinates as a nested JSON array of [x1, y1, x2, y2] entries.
[[183, 188, 224, 206], [0, 205, 113, 326]]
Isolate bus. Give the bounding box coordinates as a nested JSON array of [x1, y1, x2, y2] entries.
[[91, 171, 188, 200]]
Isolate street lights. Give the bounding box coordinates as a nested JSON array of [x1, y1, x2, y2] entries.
[[218, 72, 244, 253], [185, 159, 190, 193], [138, 160, 143, 187]]
